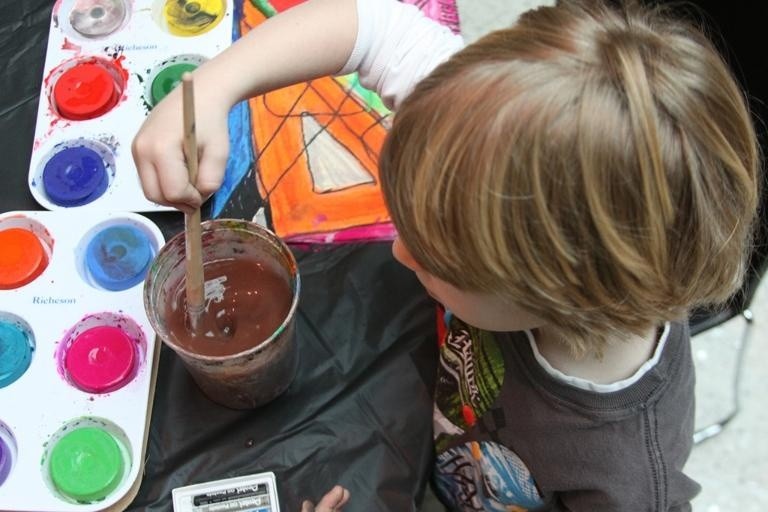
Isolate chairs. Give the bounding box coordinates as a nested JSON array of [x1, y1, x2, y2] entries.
[[608, 1, 768, 448]]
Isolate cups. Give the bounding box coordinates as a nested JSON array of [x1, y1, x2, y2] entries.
[[143, 219, 302, 411]]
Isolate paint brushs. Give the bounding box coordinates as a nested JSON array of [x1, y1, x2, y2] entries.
[[180, 71, 206, 329]]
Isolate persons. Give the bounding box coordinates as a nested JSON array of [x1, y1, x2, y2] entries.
[[131, 0, 759, 512]]
